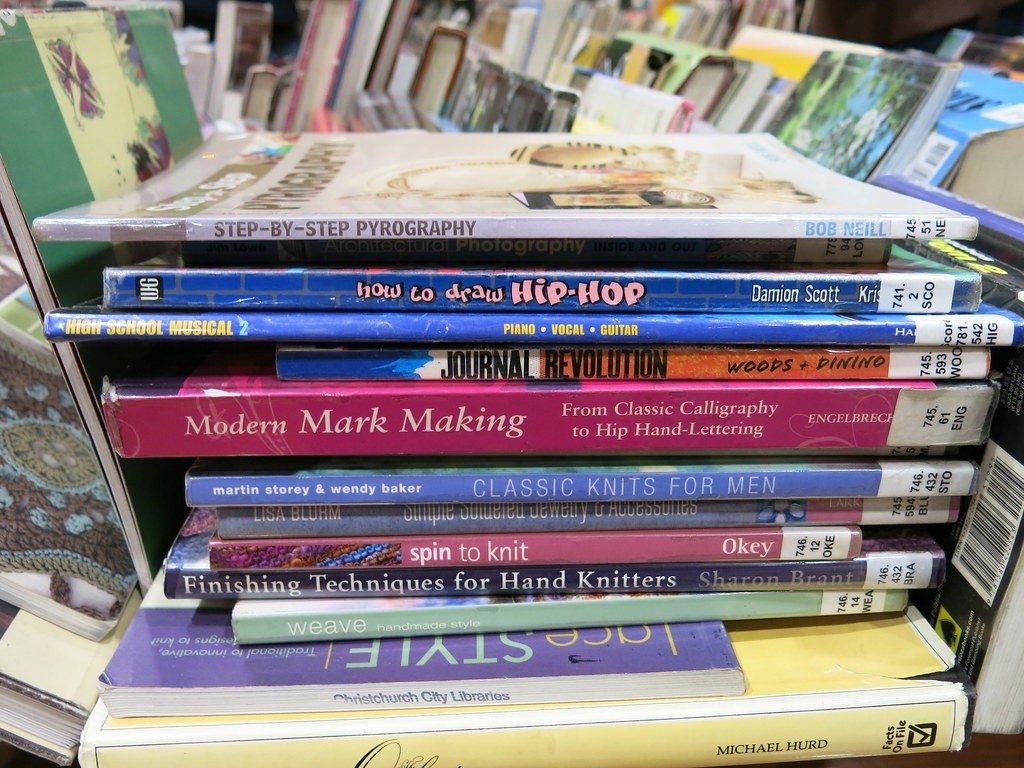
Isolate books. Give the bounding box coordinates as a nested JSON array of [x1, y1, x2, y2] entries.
[[0, 0, 1024, 768]]
